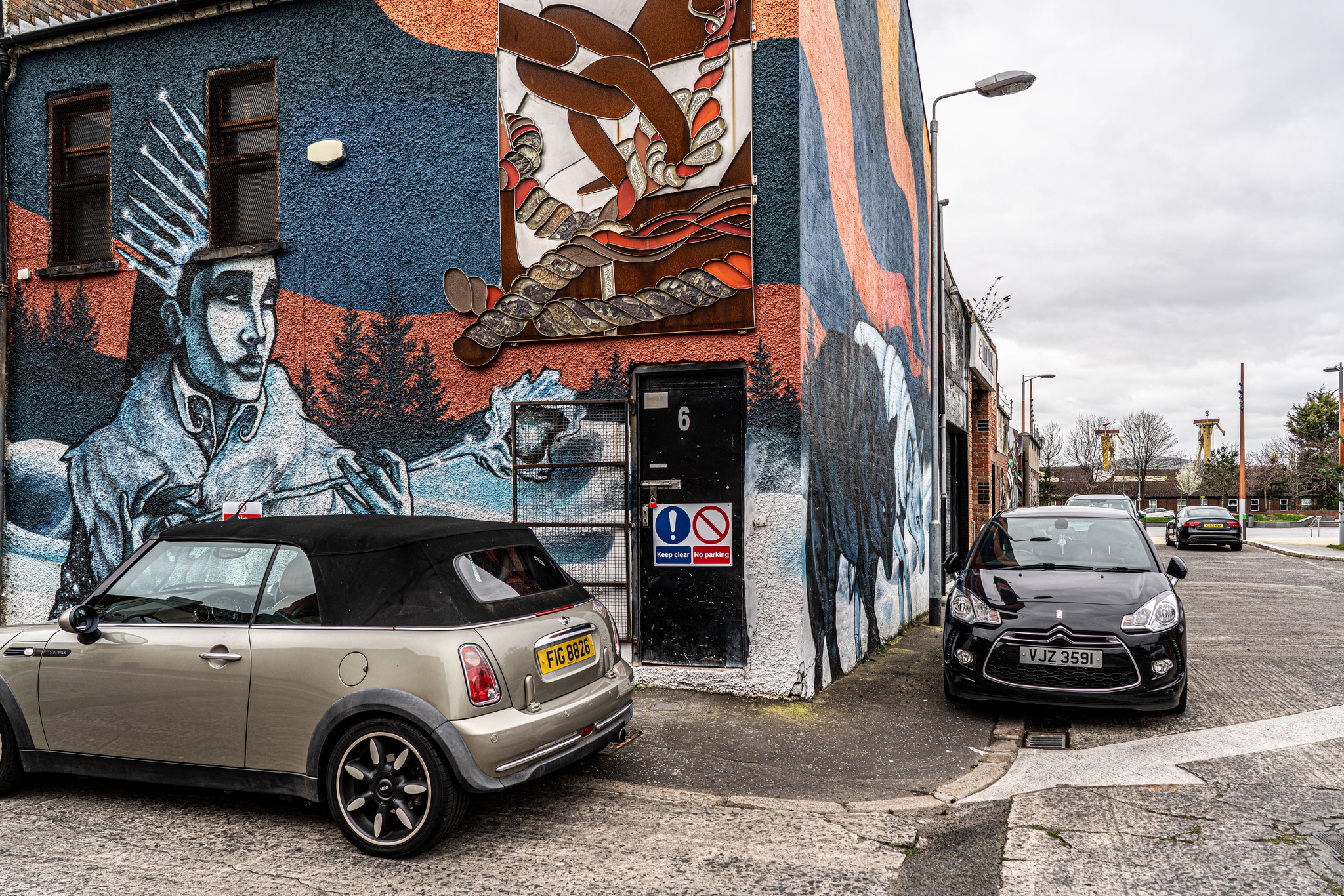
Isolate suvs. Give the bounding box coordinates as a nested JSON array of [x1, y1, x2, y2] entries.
[[1064, 493, 1147, 528]]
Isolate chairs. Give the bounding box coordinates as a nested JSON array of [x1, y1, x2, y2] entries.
[[1003, 526, 1041, 562], [271, 555, 316, 611], [1111, 503, 1121, 509], [1078, 523, 1129, 563]]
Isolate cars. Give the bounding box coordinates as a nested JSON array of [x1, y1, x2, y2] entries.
[[1137, 507, 1175, 518], [1165, 505, 1243, 552], [0, 513, 643, 858], [944, 505, 1188, 716]]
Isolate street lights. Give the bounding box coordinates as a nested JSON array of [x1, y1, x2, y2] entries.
[[927, 68, 1038, 627], [1021, 374, 1057, 431], [1322, 362, 1344, 546], [1138, 444, 1144, 511]]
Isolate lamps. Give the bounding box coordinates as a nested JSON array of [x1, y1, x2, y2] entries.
[[307, 138, 347, 167], [949, 286, 959, 296]]
